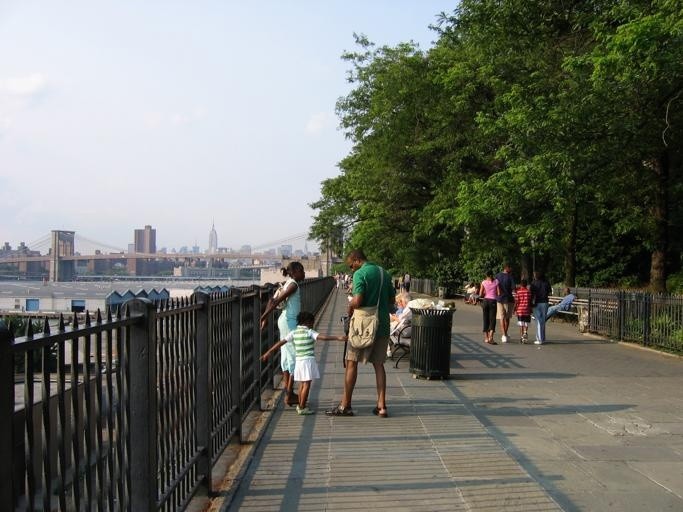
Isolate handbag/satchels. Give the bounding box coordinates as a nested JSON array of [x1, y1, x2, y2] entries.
[[348, 305, 379, 350]]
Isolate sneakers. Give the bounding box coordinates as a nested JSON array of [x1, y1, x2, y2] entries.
[[284, 394, 299, 407], [520, 333, 528, 344], [534, 341, 544, 344], [502, 335, 510, 342], [484, 336, 496, 344], [296, 404, 316, 415]]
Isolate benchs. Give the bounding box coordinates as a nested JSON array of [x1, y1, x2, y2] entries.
[[548, 296, 618, 334], [454, 288, 484, 305], [390, 326, 413, 369]]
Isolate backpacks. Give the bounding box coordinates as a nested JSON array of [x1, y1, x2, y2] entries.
[[273, 282, 287, 310]]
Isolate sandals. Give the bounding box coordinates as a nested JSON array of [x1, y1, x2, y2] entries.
[[373, 406, 388, 417], [325, 405, 353, 416]]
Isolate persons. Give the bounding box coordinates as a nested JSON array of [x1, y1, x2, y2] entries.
[[513, 279, 531, 344], [463, 282, 475, 304], [528, 267, 552, 345], [321, 249, 395, 416], [258, 261, 308, 407], [331, 269, 413, 357], [257, 311, 348, 416], [495, 264, 516, 344], [479, 269, 501, 345], [465, 282, 480, 305], [531, 286, 575, 323]]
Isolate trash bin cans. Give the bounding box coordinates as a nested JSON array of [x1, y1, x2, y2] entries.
[[438, 287, 446, 299], [408, 308, 456, 379]]
